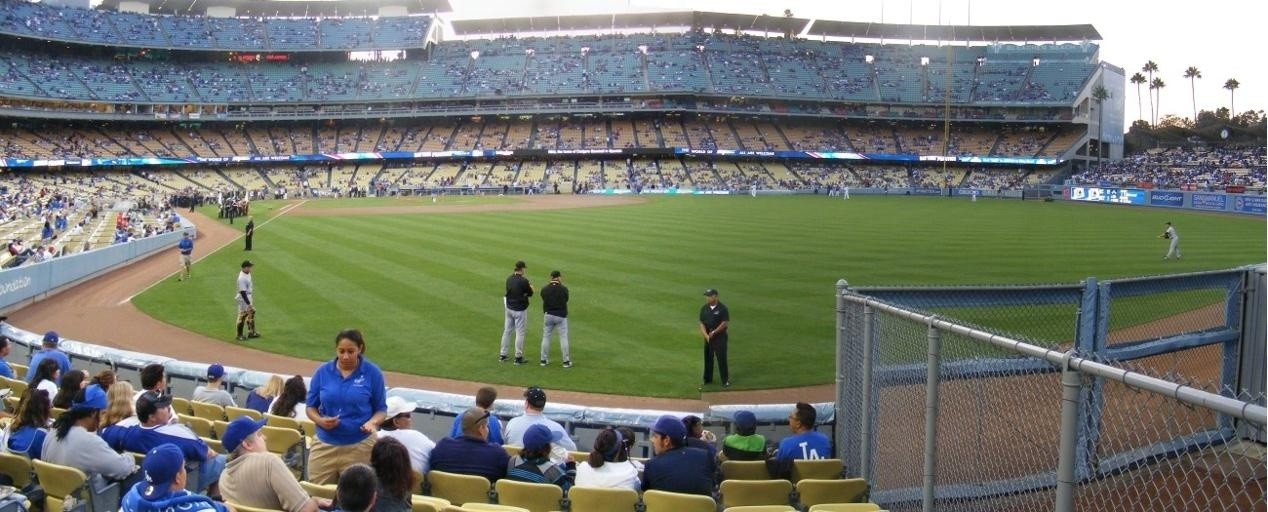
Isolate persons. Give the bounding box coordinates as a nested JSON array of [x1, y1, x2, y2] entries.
[[0, 0, 1267, 511]]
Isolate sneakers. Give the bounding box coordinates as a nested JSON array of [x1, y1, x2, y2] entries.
[[236, 336, 246, 341], [563, 361, 572, 368], [540, 360, 551, 366], [499, 355, 512, 361], [248, 332, 260, 338], [514, 356, 529, 365]]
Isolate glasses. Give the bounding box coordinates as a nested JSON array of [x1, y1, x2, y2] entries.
[[475, 410, 490, 424]]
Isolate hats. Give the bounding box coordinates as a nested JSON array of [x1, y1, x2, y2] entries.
[[516, 261, 527, 268], [385, 396, 418, 421], [704, 289, 718, 297], [649, 414, 688, 438], [137, 443, 183, 502], [222, 415, 267, 454], [208, 365, 227, 377], [136, 390, 173, 415], [523, 424, 563, 446], [242, 260, 253, 267], [43, 332, 58, 343], [523, 387, 546, 408], [734, 410, 756, 429]]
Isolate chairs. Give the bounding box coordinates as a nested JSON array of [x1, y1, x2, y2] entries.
[[0, 358, 881, 512]]
